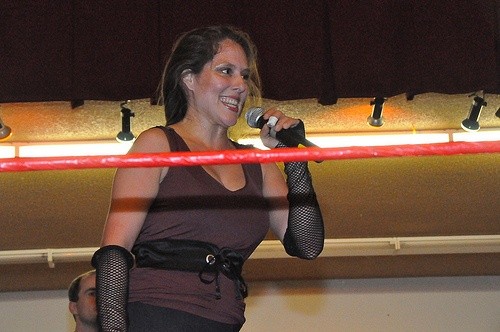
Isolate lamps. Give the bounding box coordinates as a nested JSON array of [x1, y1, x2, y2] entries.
[[366, 97, 386, 127], [461, 96, 487, 133]]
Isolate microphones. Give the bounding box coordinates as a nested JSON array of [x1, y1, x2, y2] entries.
[[245, 105, 322, 164]]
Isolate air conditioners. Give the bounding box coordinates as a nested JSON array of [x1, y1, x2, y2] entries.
[[115, 108, 135, 144]]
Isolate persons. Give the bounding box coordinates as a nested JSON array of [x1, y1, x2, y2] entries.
[[69, 270, 105, 332], [89, 24, 325, 332]]
